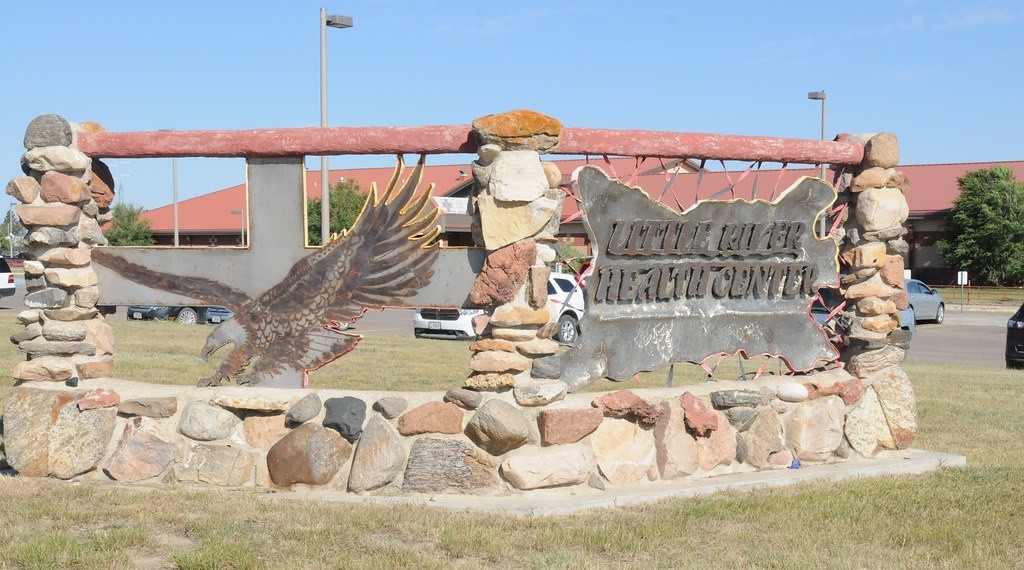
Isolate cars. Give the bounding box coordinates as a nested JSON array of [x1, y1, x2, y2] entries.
[[1005, 302, 1024, 368], [127, 305, 233, 325], [0, 253, 16, 297], [811, 278, 945, 365]]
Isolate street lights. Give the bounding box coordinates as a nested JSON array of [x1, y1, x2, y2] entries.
[[10, 203, 17, 259], [808, 90, 827, 237], [320, 7, 353, 247]]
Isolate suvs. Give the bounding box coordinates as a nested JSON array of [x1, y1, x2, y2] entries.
[[414, 272, 589, 344]]
